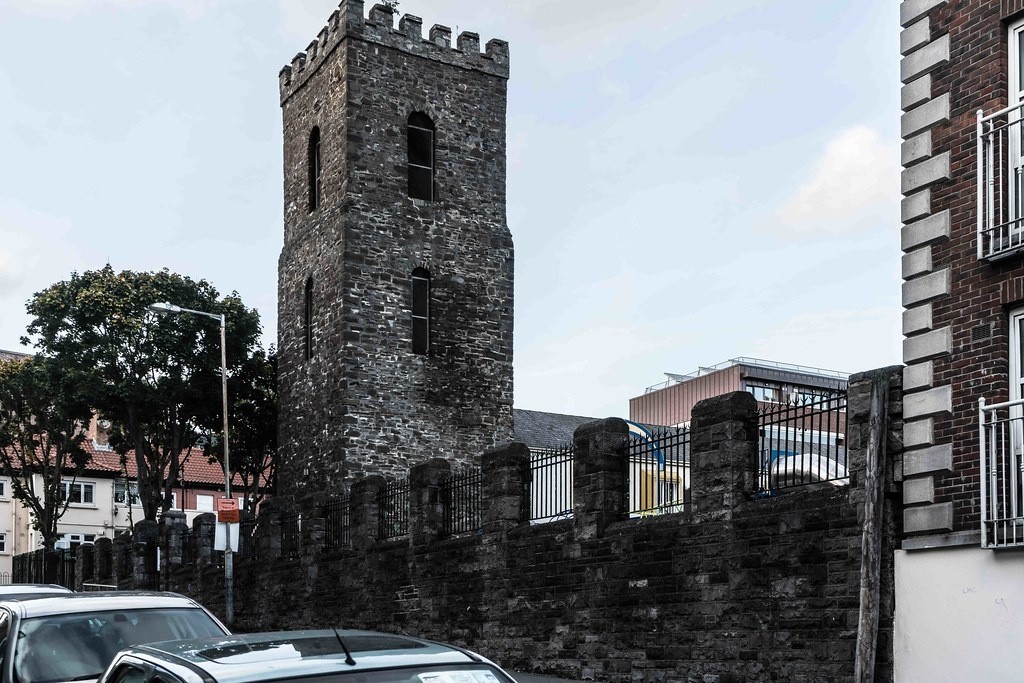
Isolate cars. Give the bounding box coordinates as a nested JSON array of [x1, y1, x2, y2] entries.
[[93, 628, 520, 683]]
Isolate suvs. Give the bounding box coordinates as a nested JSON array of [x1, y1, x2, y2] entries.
[[0, 590, 233, 683], [0, 583, 73, 601]]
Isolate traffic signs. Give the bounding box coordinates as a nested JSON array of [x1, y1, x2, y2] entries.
[[217, 498, 240, 523]]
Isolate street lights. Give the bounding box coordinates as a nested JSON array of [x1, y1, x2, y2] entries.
[[148, 301, 234, 634]]
